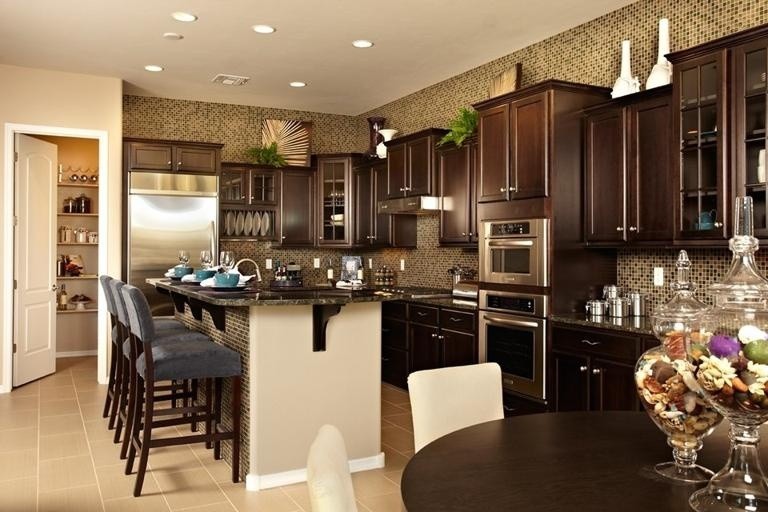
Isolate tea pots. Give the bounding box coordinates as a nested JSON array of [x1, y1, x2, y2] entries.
[[697, 209, 719, 231]]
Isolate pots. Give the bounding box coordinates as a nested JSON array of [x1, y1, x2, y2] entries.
[[584, 284, 646, 318], [584, 314, 645, 329]]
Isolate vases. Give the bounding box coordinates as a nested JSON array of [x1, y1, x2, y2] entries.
[[360, 115, 386, 166], [611, 40, 641, 99], [646, 18, 673, 90]]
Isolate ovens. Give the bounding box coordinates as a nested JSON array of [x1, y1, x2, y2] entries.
[[477, 290, 549, 405]]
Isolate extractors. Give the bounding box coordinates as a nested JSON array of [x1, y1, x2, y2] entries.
[[377, 196, 454, 215]]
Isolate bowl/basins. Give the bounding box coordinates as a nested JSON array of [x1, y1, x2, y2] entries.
[[174, 267, 194, 277], [214, 273, 239, 287], [330, 213, 344, 223], [195, 269, 217, 280]]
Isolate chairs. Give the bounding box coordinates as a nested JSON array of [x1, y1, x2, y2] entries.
[[407, 362, 504, 454], [306, 423, 357, 512], [100, 275, 242, 497]]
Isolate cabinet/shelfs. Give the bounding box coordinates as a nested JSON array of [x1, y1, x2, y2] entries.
[[220, 128, 478, 250], [472, 78, 611, 221], [381, 301, 479, 391], [664, 22, 768, 251], [582, 82, 673, 250], [551, 323, 642, 414], [121, 137, 224, 176], [56, 182, 98, 358]]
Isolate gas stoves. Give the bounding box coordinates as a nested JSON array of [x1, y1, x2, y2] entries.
[[388, 285, 450, 298]]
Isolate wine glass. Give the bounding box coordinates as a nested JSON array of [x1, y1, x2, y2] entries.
[[219, 251, 236, 273], [177, 251, 191, 267], [199, 250, 212, 270], [327, 187, 346, 205]]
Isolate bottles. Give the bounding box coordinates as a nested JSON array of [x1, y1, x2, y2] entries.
[[57, 261, 64, 277], [326, 259, 334, 283], [685, 195, 768, 512], [635, 247, 725, 483], [373, 264, 397, 295], [57, 283, 68, 310], [273, 259, 301, 281], [356, 258, 364, 283]]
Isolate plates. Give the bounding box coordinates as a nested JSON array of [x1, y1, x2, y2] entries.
[[210, 284, 251, 291], [220, 211, 271, 238], [180, 274, 200, 284], [168, 274, 192, 280]]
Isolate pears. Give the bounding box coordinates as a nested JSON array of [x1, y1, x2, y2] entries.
[[738, 325, 768, 365]]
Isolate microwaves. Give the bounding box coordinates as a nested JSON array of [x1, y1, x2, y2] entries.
[[477, 218, 550, 288]]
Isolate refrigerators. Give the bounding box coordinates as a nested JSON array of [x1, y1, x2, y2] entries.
[[125, 171, 218, 316]]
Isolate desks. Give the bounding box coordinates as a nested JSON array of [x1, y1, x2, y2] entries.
[[401, 411, 768, 512]]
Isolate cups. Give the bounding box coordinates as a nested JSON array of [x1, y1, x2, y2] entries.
[[88, 231, 97, 243]]
